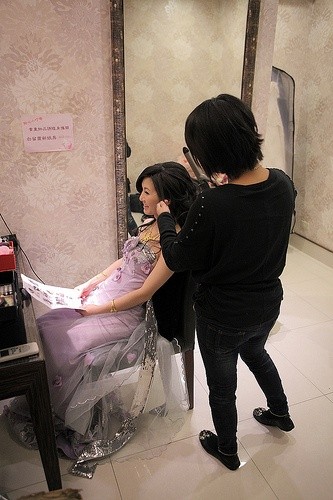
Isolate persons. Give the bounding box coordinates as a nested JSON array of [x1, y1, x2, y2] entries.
[[127, 141, 140, 236], [2, 162, 217, 483], [156, 94, 298, 471]]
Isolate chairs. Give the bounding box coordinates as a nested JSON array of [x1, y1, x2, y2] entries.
[[83, 179, 209, 435]]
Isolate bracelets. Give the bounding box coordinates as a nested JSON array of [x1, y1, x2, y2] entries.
[[101, 272, 108, 278], [111, 299, 118, 312]]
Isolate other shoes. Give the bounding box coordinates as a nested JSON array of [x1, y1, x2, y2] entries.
[[252, 407, 294, 432], [199, 430, 240, 470]]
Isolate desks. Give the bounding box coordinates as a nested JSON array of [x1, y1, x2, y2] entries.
[[0, 238, 62, 494]]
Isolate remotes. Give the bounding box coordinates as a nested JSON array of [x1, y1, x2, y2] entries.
[[0, 342, 39, 363]]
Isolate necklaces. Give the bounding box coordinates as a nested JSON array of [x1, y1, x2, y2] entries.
[[144, 222, 160, 240]]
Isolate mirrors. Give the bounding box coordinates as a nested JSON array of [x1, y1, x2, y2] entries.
[[110, 0, 261, 261]]
